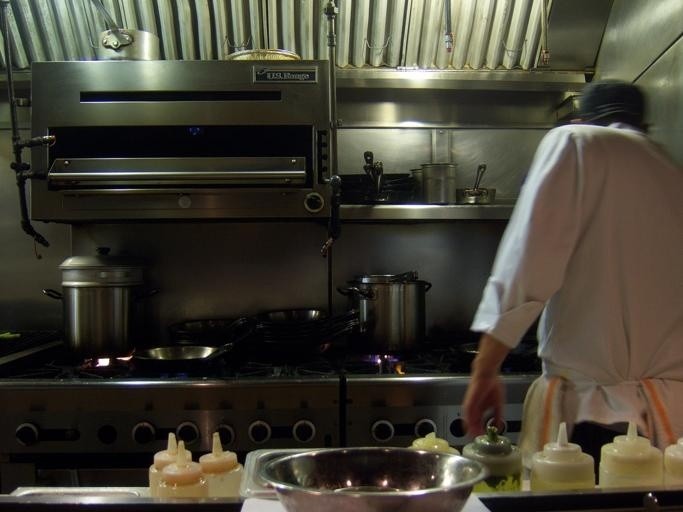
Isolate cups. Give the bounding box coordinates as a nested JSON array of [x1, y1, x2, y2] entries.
[[414, 160, 457, 204]]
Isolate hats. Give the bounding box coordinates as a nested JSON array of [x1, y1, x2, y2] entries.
[[579, 80, 643, 124]]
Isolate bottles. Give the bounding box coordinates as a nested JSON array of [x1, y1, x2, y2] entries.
[[157, 441, 209, 497], [599, 420, 664, 488], [665, 436, 683, 491], [407, 432, 459, 454], [462, 426, 523, 491], [530, 422, 595, 489], [148, 431, 192, 496], [198, 432, 244, 497]]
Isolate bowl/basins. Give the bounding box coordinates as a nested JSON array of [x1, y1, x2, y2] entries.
[[258, 445, 490, 512]]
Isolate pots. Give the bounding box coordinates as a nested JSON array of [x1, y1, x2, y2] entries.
[[91, 0, 161, 61], [132, 342, 234, 367], [335, 269, 431, 359], [339, 149, 412, 203], [432, 324, 537, 372], [41, 246, 161, 361], [456, 163, 497, 205]]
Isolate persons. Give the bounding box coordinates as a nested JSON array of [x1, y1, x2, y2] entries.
[[461, 79, 683, 486]]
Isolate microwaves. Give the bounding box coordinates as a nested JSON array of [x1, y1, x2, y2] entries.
[[29, 58, 335, 221]]
